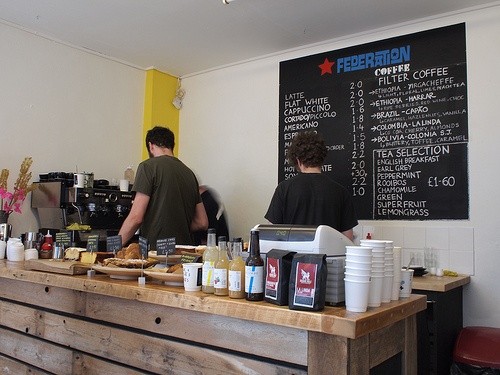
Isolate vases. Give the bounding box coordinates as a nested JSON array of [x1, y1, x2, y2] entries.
[[0, 210, 10, 224]]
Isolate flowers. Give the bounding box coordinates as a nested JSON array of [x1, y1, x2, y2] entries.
[[0, 188, 27, 213]]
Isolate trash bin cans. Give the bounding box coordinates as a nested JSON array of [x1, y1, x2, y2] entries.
[[452, 327, 500, 375]]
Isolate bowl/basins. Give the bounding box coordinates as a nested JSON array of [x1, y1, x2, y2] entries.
[[402, 265, 427, 276], [92, 250, 183, 286]]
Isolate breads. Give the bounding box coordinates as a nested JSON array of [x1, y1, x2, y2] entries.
[[65, 243, 210, 275]]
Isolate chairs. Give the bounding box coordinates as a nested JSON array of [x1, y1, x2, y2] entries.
[[453, 324, 500, 375]]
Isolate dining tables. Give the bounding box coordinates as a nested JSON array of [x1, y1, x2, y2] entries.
[[0, 262, 470, 375]]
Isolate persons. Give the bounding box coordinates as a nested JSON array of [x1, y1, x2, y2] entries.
[[192, 185, 229, 246], [264, 131, 358, 240], [118, 127, 208, 251]]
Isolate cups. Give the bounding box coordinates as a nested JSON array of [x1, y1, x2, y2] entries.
[[1, 223, 64, 262], [430, 267, 444, 277], [183, 262, 204, 292], [73, 172, 94, 188], [120, 179, 131, 192], [343, 240, 414, 312]]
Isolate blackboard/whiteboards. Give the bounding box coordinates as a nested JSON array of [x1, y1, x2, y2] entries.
[[278, 22, 469, 220]]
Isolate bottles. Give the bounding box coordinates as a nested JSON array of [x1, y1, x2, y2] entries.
[[124, 167, 134, 186], [202, 228, 246, 298], [45, 230, 53, 244], [244, 230, 265, 300]]
